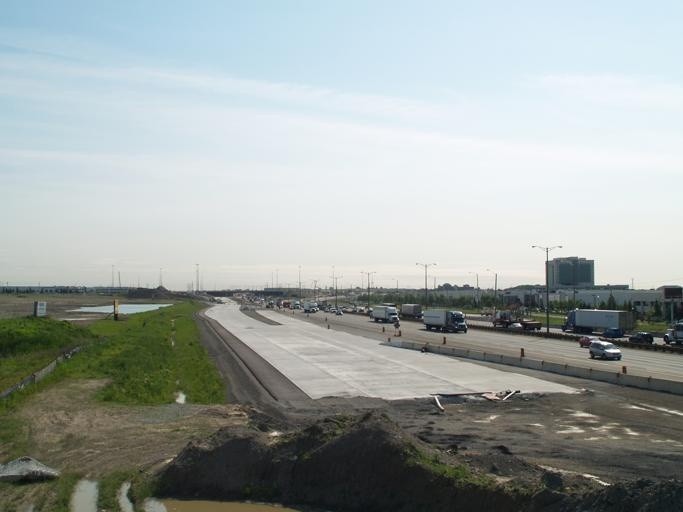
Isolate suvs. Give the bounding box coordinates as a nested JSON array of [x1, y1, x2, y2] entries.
[[589, 341, 622, 360], [629, 332, 653, 344]]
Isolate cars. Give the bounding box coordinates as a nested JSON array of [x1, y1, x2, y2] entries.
[[580, 335, 601, 348]]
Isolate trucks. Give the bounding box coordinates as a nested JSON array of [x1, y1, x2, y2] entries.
[[424, 308, 468, 334], [372, 305, 400, 323], [663, 322, 683, 345], [562, 308, 633, 338], [402, 303, 423, 319]]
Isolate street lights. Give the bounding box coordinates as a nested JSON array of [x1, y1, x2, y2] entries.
[[531, 245, 563, 332], [416, 263, 437, 310], [361, 271, 377, 310]]
